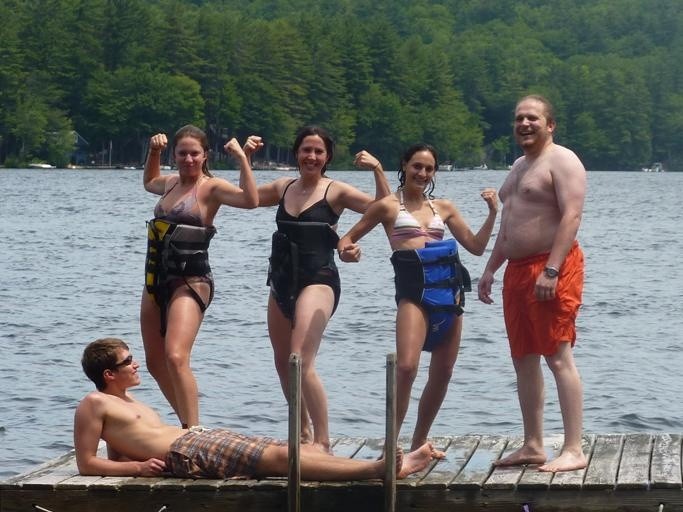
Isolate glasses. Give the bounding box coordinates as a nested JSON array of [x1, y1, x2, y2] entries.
[[115, 353, 133, 366]]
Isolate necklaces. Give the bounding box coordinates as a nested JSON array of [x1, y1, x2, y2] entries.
[[301, 184, 309, 196]]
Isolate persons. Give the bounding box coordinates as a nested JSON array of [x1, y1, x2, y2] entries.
[[136, 123, 260, 427], [71, 337, 438, 483], [476, 91, 590, 476], [331, 144, 499, 465], [236, 123, 391, 455]]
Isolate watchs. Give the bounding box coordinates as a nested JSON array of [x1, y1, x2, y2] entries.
[[543, 265, 561, 280]]
